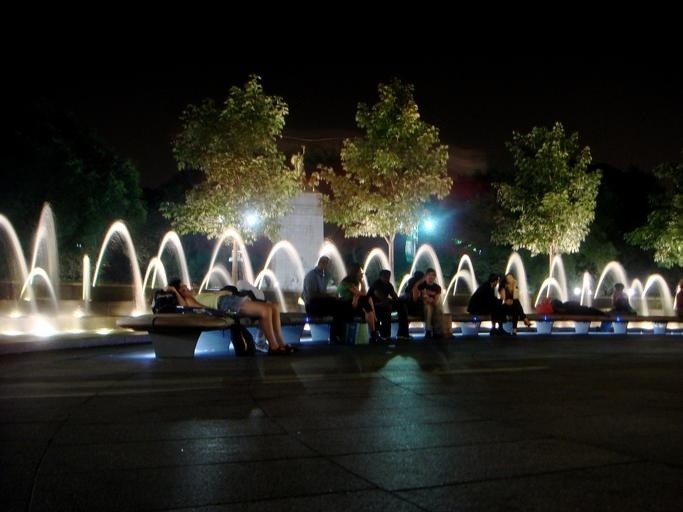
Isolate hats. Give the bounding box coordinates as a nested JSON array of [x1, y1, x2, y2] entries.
[[490, 328, 518, 337]]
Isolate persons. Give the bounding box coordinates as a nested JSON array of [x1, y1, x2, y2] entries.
[[366, 268, 413, 341], [168, 277, 300, 357], [499, 272, 532, 336], [549, 299, 610, 316], [597, 284, 630, 332], [466, 270, 511, 336], [399, 267, 443, 337], [302, 255, 350, 345], [674, 279, 683, 317], [335, 262, 387, 344]]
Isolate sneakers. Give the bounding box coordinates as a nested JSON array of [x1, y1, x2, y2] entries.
[[370, 335, 412, 342], [256, 344, 297, 355]]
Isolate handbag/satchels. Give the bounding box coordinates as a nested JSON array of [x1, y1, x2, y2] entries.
[[536, 297, 553, 314], [230, 324, 255, 356], [155, 290, 177, 314], [345, 323, 369, 345]]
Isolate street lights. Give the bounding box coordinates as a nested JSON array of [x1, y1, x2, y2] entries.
[[412, 210, 435, 261]]
